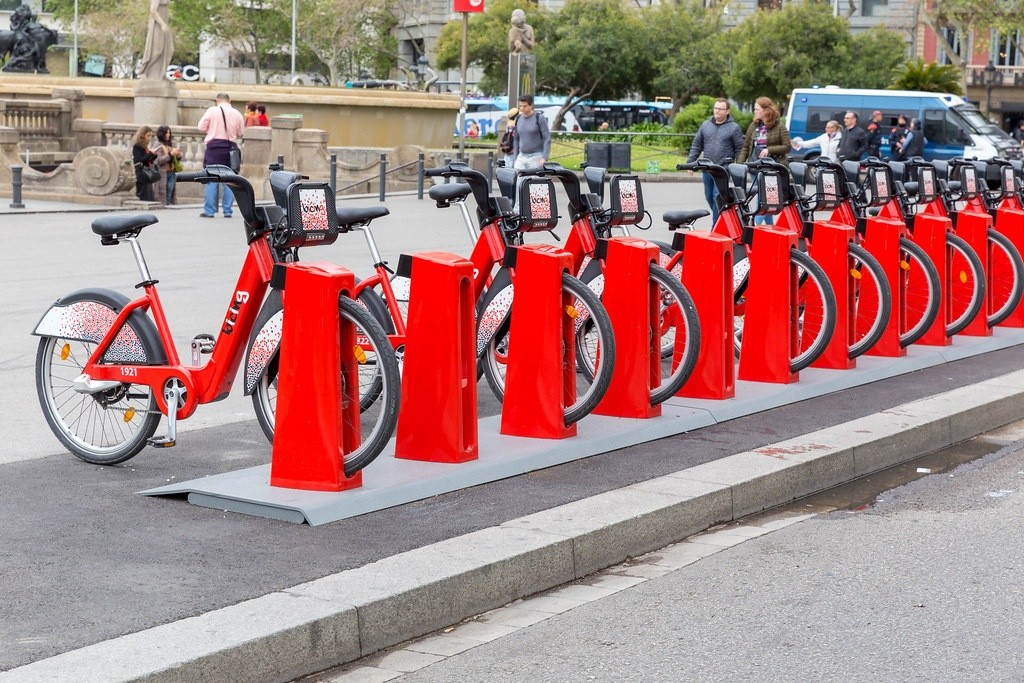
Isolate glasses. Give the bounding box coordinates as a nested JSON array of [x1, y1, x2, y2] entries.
[[712, 107, 727, 112]]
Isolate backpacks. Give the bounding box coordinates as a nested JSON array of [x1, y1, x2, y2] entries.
[[499, 122, 516, 153]]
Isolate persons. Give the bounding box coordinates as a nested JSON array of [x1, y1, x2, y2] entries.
[[465, 121, 479, 139], [198, 93, 244, 218], [598, 122, 608, 131], [137, 0, 174, 82], [737, 97, 791, 225], [2, 4, 55, 72], [797, 110, 868, 204], [867, 111, 882, 158], [686, 98, 744, 223], [504, 94, 551, 169], [888, 114, 927, 181], [132, 125, 183, 205], [244, 102, 268, 126], [1012, 119, 1024, 145], [509, 8, 535, 54]]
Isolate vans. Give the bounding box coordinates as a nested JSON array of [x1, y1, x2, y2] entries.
[[785, 87, 1023, 185]]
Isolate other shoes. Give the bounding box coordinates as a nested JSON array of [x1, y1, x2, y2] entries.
[[224, 215, 231, 218], [200, 212, 214, 218]]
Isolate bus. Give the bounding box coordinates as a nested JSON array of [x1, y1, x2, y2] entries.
[[460, 96, 673, 133]]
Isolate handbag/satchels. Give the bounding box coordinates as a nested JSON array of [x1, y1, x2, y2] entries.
[[229, 148, 241, 174], [173, 155, 183, 172], [142, 164, 161, 183], [746, 146, 778, 175]]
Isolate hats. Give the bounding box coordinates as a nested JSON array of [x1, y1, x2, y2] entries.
[[508, 107, 524, 127]]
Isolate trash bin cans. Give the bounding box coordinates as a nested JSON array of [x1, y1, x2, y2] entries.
[[584, 143, 609, 170], [608, 143, 631, 174]]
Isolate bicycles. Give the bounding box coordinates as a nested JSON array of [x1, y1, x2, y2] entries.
[[31, 163, 400, 472], [271, 158, 1023, 426]]
